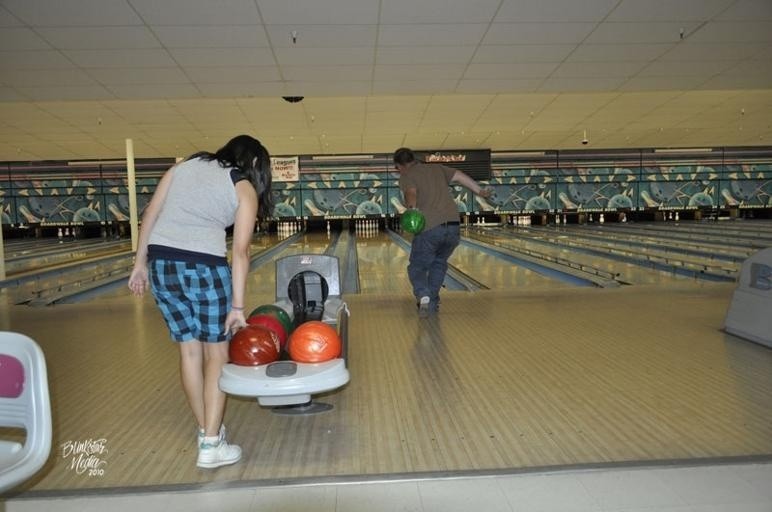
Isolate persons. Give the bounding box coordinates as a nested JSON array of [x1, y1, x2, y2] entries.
[[393, 147, 492, 313], [128, 135, 271, 471]]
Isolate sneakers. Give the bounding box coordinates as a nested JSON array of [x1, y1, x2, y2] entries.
[[419, 295, 440, 318], [195, 424, 242, 469]]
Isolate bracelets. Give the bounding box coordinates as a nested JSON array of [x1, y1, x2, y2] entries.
[[230, 303, 246, 312]]
